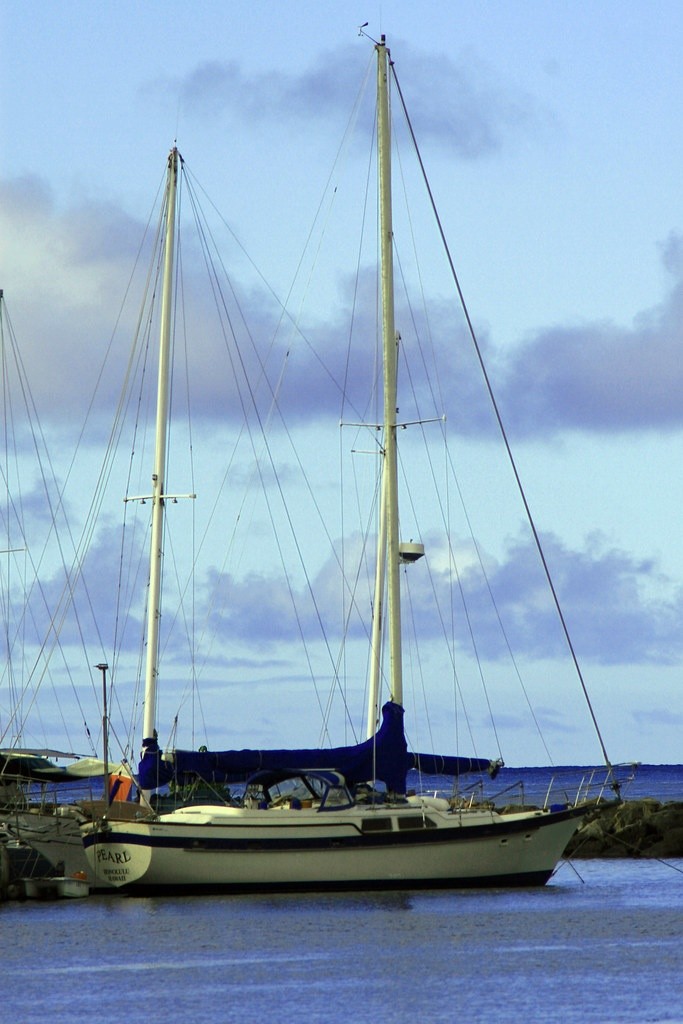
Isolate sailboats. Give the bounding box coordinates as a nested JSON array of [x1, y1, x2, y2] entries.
[[0, 16, 645, 907]]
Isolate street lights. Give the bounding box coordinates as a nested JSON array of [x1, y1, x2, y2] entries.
[[97, 663, 110, 811]]
